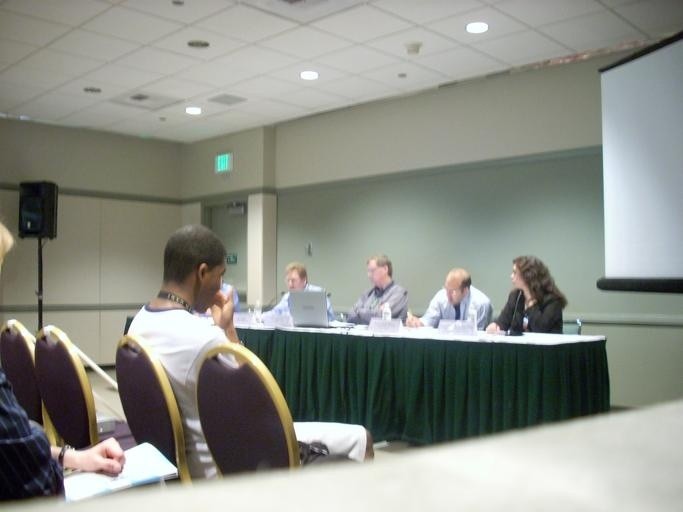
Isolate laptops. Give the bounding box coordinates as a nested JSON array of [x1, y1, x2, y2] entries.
[[291, 291, 354, 328]]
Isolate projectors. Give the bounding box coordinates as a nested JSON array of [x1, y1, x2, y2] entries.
[[95, 409, 117, 435]]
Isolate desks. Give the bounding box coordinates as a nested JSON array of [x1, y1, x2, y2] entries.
[[219, 318, 611, 447]]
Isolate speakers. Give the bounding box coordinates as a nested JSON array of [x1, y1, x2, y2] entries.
[[18, 180, 59, 240]]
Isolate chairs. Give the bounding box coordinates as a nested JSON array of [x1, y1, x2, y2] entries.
[[195, 342, 301, 475], [36, 325, 134, 452], [115, 335, 191, 485], [2, 317, 54, 442]]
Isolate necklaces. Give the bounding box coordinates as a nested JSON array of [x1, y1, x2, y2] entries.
[[156, 289, 193, 315]]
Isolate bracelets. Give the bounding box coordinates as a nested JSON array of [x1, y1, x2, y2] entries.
[[57, 443, 77, 471], [527, 297, 537, 307]]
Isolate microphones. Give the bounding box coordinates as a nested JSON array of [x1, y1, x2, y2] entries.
[[262, 291, 285, 315], [327, 292, 332, 298], [390, 290, 408, 310], [505, 290, 524, 336]]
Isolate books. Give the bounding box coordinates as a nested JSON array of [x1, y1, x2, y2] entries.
[[59, 440, 179, 504]]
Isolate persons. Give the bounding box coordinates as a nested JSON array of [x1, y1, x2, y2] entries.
[[260, 262, 335, 323], [485, 255, 567, 335], [0, 221, 124, 501], [405, 268, 491, 328], [125, 224, 374, 477], [193, 282, 240, 316], [338, 253, 408, 326]]
